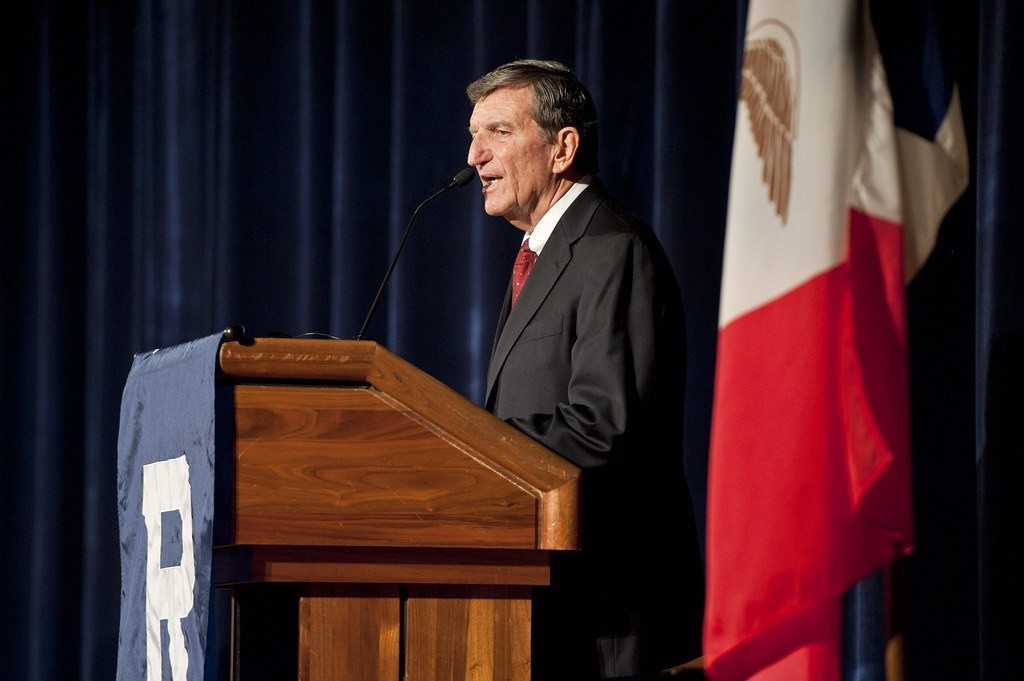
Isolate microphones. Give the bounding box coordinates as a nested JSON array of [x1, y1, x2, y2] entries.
[[356, 168, 475, 341]]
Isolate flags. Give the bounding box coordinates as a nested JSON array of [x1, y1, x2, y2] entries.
[[699, 0, 967, 680]]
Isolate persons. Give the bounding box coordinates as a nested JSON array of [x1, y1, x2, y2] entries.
[[468, 57, 683, 681]]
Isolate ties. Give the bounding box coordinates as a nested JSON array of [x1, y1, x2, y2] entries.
[[512, 237, 538, 311]]
